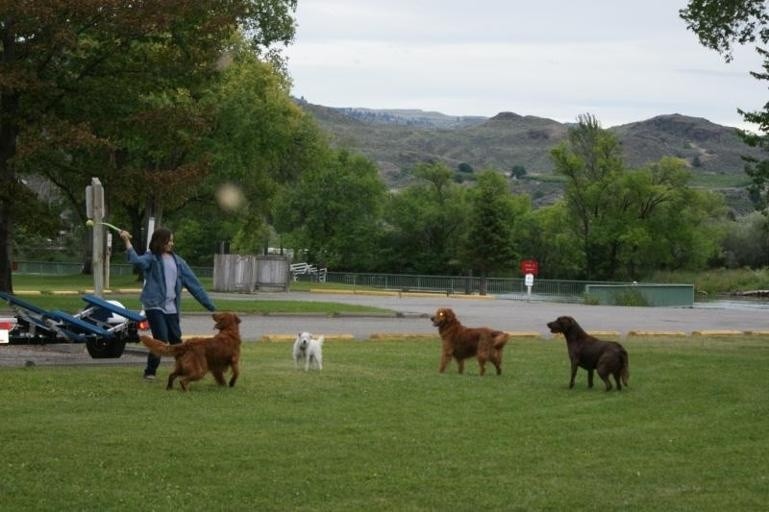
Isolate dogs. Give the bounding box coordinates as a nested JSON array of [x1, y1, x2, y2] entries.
[[545, 314, 630, 392], [137, 311, 244, 397], [429, 306, 510, 378], [289, 330, 330, 372]]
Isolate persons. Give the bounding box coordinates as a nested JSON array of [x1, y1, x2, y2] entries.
[[117, 226, 217, 381]]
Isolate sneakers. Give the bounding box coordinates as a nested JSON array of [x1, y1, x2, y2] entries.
[[144, 372, 157, 381]]
